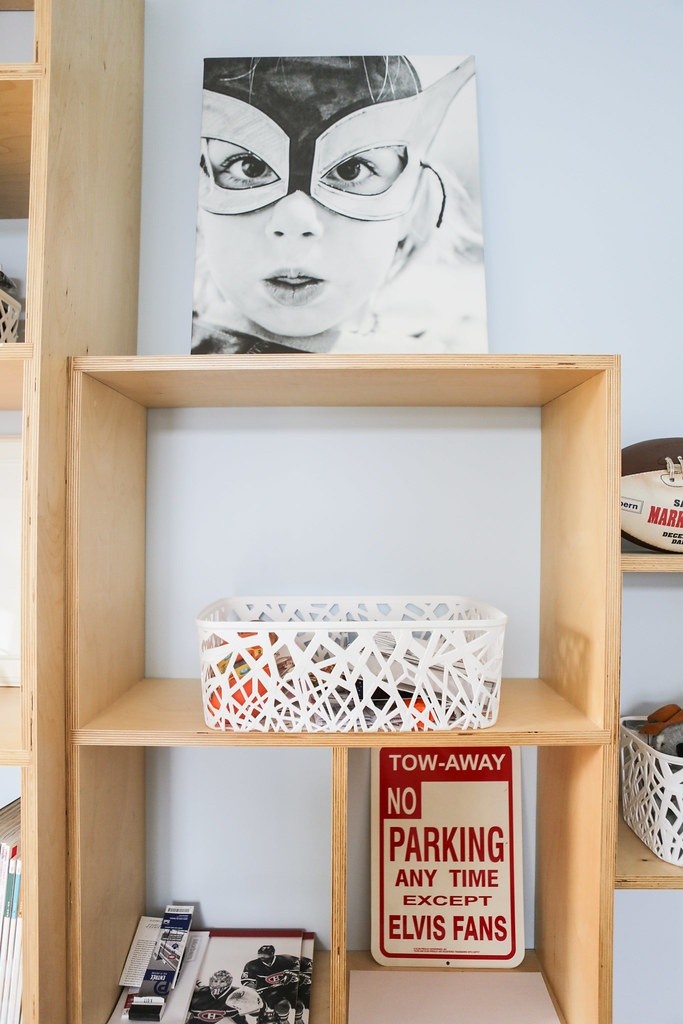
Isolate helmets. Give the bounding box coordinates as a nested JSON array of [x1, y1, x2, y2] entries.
[[209, 969, 233, 998], [258, 945, 275, 954], [225, 986, 263, 1016]]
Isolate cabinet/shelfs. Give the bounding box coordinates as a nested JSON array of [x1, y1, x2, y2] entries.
[[0, 0, 683, 1024]]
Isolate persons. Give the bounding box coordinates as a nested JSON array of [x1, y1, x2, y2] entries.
[[192, 57, 446, 359], [187, 945, 312, 1024]]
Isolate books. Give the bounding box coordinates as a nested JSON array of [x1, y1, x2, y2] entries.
[[0, 797, 23, 1024]]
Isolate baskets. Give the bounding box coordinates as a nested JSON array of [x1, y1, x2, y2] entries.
[[619, 715, 683, 867], [195, 594, 509, 734]]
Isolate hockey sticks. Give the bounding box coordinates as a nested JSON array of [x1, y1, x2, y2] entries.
[[257, 983, 282, 992]]
